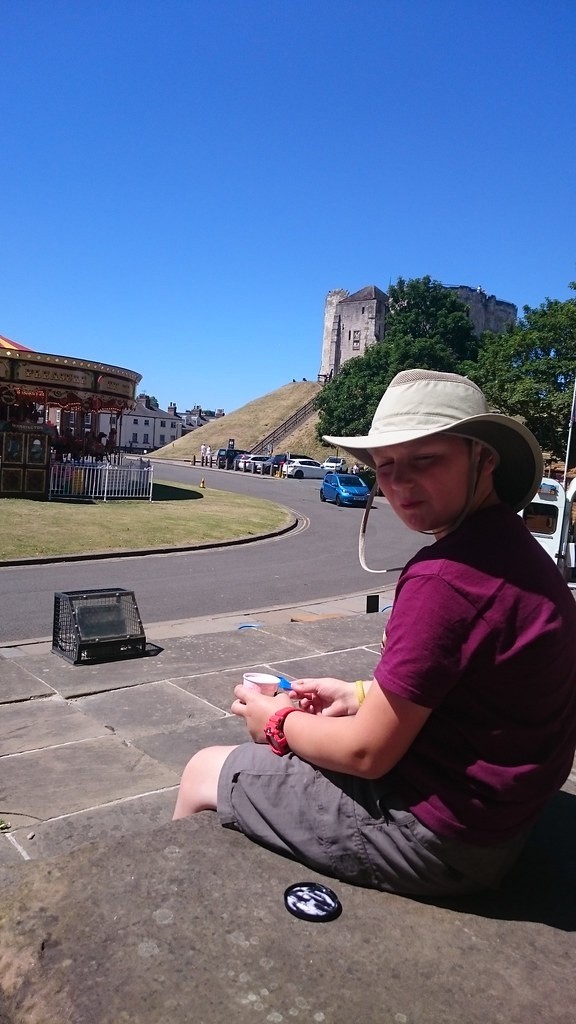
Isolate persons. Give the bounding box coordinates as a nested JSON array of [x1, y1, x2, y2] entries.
[[201, 443, 211, 462], [353, 463, 360, 474], [172, 369, 576, 897], [340, 460, 348, 474]]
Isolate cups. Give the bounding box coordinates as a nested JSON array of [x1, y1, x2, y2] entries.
[[243, 673, 280, 697]]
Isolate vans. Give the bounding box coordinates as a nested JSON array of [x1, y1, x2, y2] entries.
[[272, 454, 313, 475], [217, 449, 249, 468], [282, 457, 325, 479], [234, 454, 271, 472]]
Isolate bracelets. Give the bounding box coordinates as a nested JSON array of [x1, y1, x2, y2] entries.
[[355, 680, 365, 706]]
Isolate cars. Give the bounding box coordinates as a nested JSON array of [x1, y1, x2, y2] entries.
[[320, 474, 372, 507], [262, 457, 275, 474]]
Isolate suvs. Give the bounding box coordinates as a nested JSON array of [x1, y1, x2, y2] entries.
[[322, 456, 348, 474]]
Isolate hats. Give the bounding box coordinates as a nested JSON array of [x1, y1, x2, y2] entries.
[[322, 369, 544, 514]]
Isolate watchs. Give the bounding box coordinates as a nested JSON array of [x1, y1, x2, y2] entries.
[[263, 707, 306, 757]]
